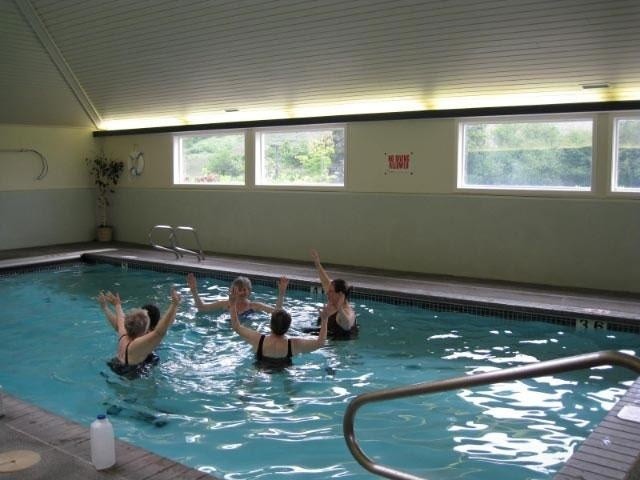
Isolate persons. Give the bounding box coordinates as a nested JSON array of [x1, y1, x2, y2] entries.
[[95, 290, 161, 365], [103, 283, 183, 380], [185, 270, 291, 319], [227, 285, 331, 375], [308, 245, 357, 341]]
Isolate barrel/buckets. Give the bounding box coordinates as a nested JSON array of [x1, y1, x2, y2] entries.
[[90, 415, 116, 469]]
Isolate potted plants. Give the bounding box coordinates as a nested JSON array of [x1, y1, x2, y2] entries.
[[84, 152, 125, 244]]
